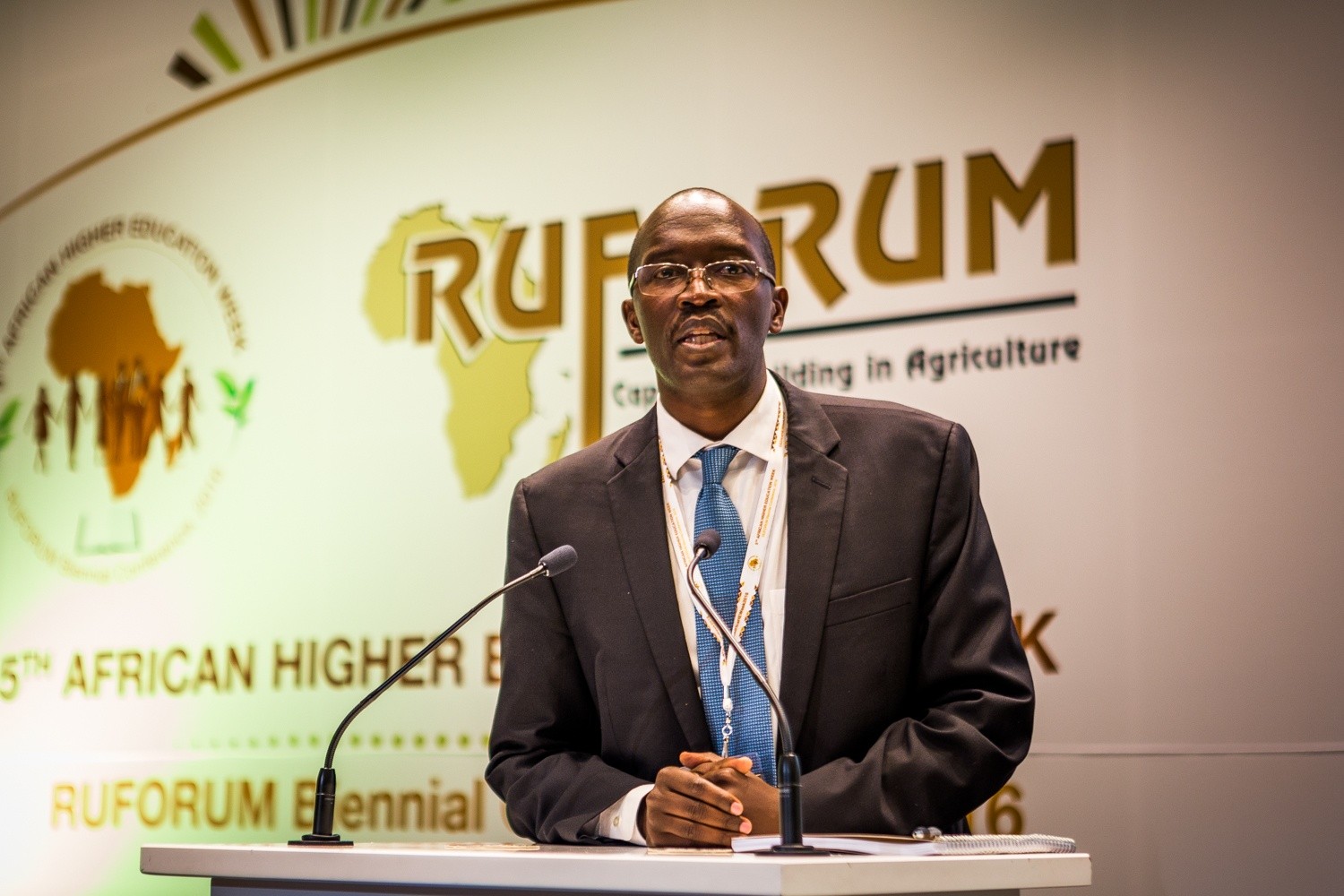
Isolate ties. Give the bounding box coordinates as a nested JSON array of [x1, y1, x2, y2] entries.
[[689, 445, 779, 789]]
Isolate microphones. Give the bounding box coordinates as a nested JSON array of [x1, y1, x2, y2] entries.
[[314, 545, 580, 836], [687, 528, 806, 847]]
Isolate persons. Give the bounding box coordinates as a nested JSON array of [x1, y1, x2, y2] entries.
[[487, 188, 1036, 846]]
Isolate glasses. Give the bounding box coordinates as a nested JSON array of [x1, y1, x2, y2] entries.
[[628, 259, 776, 297]]
